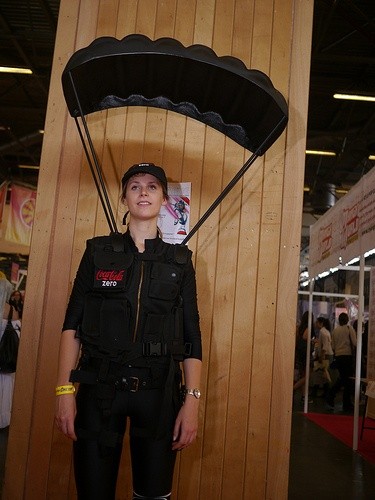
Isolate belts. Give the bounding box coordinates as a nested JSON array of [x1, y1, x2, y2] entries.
[[102, 376, 164, 392]]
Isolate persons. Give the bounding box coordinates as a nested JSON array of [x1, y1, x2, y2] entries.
[[55, 163, 202, 500], [293, 309, 368, 415], [0, 259, 22, 500]]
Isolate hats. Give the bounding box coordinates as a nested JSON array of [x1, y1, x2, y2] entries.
[[121, 163, 167, 189]]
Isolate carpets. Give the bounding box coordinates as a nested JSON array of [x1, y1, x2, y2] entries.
[[305, 412, 375, 466]]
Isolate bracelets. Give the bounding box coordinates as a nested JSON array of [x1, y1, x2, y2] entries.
[[55, 384, 75, 396]]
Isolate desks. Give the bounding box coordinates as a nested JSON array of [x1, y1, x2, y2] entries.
[[350, 377, 375, 441]]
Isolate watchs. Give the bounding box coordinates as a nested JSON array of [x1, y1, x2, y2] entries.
[[185, 388, 201, 399]]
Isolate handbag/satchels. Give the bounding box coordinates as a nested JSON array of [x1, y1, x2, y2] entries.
[[0, 291, 24, 374]]
